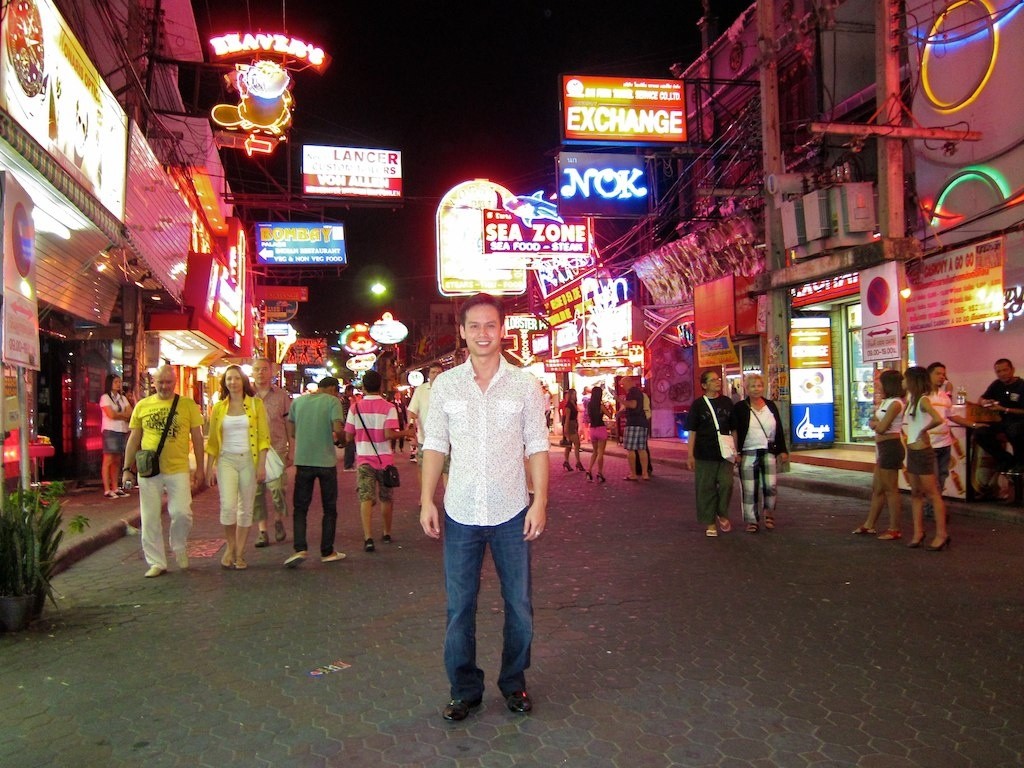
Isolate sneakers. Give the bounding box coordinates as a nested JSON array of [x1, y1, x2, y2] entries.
[[102, 489, 131, 499], [382, 534, 391, 544], [364, 538, 374, 551]]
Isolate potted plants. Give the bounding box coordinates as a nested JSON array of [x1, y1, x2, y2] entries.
[[0, 483, 90, 631]]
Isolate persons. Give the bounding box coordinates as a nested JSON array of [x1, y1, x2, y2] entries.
[[924, 363, 989, 524], [852, 370, 904, 540], [558, 390, 569, 444], [901, 366, 950, 551], [735, 373, 789, 534], [619, 383, 652, 476], [419, 292, 550, 721], [609, 377, 650, 481], [284, 376, 347, 566], [389, 391, 408, 454], [683, 370, 738, 536], [409, 362, 450, 505], [122, 364, 204, 579], [249, 359, 294, 547], [343, 371, 416, 552], [940, 374, 953, 402], [975, 359, 1024, 506], [340, 385, 357, 471], [586, 387, 612, 482], [582, 386, 591, 442], [100, 373, 133, 499], [562, 390, 585, 471], [205, 365, 271, 571]]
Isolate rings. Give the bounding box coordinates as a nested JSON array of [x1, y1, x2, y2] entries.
[[535, 529, 541, 536], [786, 459, 789, 460], [783, 460, 786, 461]]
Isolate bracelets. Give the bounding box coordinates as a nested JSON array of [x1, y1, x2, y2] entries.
[[1004, 408, 1009, 414], [123, 467, 131, 472], [972, 422, 976, 429]]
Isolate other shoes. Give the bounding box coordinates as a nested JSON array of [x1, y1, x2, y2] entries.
[[284, 551, 306, 568], [997, 453, 1016, 472], [220, 555, 234, 569], [275, 527, 286, 542], [235, 559, 248, 569], [559, 439, 650, 482], [343, 437, 417, 473], [923, 503, 950, 520], [254, 533, 269, 547], [321, 552, 346, 563]]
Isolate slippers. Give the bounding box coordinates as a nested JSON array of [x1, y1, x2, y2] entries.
[[717, 516, 731, 532], [745, 523, 760, 535], [706, 529, 718, 537], [764, 521, 775, 531]]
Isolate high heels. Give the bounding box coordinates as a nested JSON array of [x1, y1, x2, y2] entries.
[[906, 532, 927, 548], [926, 536, 952, 551]]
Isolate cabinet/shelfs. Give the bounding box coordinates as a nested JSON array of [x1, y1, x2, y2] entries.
[[896, 403, 1000, 503]]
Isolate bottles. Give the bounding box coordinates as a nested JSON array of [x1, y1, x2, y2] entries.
[[956, 385, 967, 404]]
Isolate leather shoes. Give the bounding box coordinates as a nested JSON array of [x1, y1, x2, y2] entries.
[[145, 567, 168, 578], [506, 690, 533, 713], [176, 554, 189, 569], [442, 696, 482, 721]]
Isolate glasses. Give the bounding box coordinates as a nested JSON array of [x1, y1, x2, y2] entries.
[[707, 378, 722, 382]]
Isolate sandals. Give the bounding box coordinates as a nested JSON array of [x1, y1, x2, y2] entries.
[[878, 530, 903, 540], [852, 525, 877, 534]]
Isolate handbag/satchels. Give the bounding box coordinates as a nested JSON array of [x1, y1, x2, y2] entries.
[[768, 440, 778, 455], [384, 464, 400, 487], [136, 450, 160, 478], [717, 430, 737, 464], [261, 445, 286, 484]]
